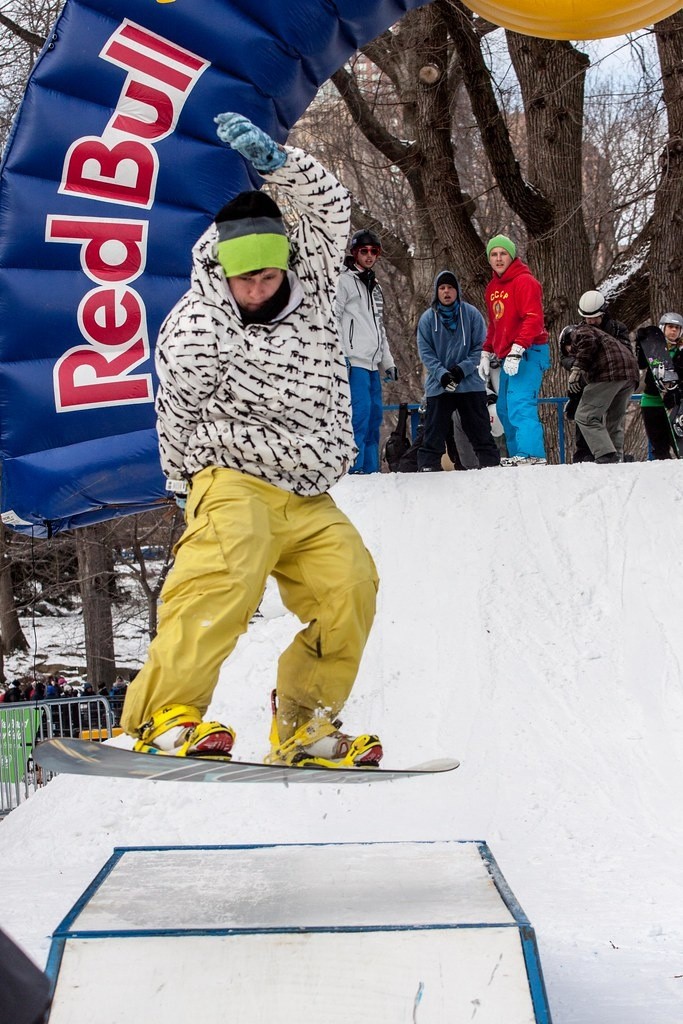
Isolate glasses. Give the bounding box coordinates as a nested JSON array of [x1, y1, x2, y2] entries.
[[358, 248, 379, 256]]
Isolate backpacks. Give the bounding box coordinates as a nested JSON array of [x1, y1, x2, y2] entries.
[[386, 432, 411, 472]]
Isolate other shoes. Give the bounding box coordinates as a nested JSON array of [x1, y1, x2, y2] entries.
[[594, 454, 620, 464]]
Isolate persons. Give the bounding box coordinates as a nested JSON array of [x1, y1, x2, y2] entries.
[[478, 235, 550, 465], [635, 312, 683, 461], [417, 271, 500, 471], [0, 676, 128, 737], [573, 291, 632, 462], [334, 230, 398, 474], [558, 325, 639, 465], [120, 111, 383, 766]]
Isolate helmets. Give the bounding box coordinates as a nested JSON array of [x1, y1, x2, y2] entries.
[[578, 290, 606, 317], [659, 313, 683, 344], [559, 325, 579, 356], [350, 229, 381, 254]]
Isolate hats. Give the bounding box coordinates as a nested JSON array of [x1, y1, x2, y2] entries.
[[486, 235, 516, 260], [437, 273, 458, 291], [215, 191, 288, 277]]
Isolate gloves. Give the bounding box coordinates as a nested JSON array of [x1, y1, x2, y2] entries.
[[385, 366, 398, 382], [478, 351, 490, 380], [442, 374, 458, 392], [503, 344, 525, 375], [448, 366, 465, 384], [568, 367, 581, 393], [213, 111, 288, 171]]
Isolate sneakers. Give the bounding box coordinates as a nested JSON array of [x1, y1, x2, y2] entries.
[[500, 457, 523, 467], [516, 455, 546, 467], [265, 689, 382, 771], [135, 704, 235, 761]]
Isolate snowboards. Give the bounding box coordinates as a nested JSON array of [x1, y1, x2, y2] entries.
[[29, 737, 467, 786], [639, 325, 683, 458]]
